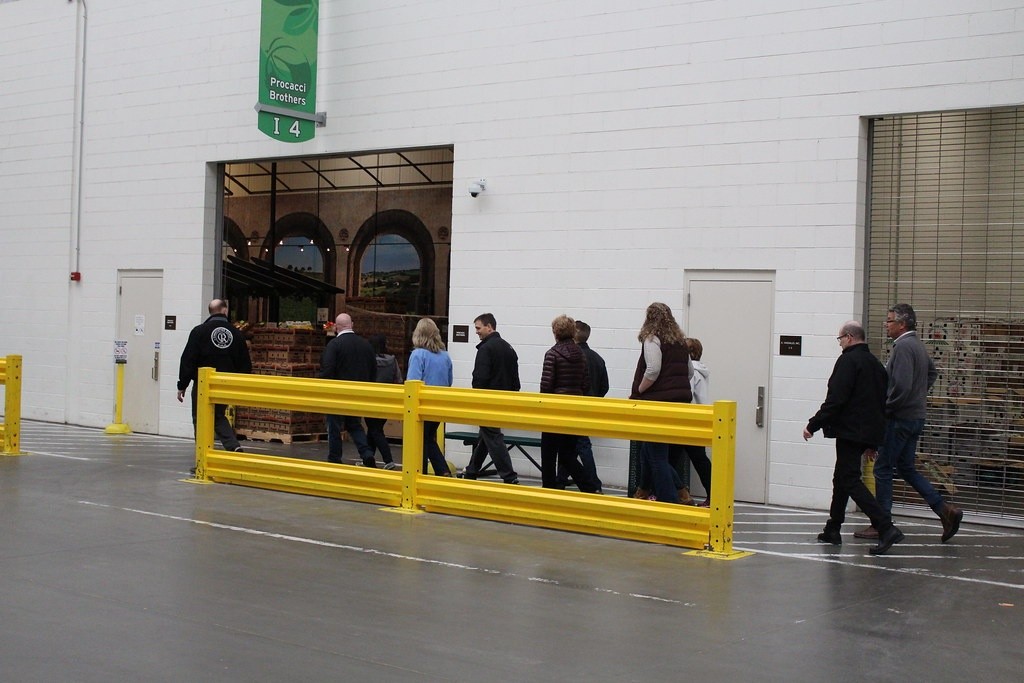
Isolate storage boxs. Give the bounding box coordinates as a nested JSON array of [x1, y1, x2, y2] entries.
[[233, 296, 449, 435]]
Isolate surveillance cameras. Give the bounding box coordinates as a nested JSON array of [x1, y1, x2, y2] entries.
[[469, 184, 482, 197]]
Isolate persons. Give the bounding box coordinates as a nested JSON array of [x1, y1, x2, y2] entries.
[[455, 313, 521, 485], [320, 313, 377, 469], [177, 298, 252, 473], [629, 302, 711, 508], [804, 319, 905, 553], [540, 314, 609, 494], [356, 334, 403, 470], [406, 318, 453, 477], [853, 304, 962, 542]]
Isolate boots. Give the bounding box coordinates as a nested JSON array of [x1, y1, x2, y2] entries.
[[633, 487, 652, 500], [676, 486, 695, 506]]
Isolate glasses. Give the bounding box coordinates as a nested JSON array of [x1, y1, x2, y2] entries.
[[884, 319, 900, 323], [837, 332, 852, 342]]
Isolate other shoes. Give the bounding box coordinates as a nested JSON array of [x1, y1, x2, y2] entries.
[[233, 446, 244, 453], [356, 461, 367, 467], [382, 462, 395, 469], [504, 474, 519, 485], [455, 472, 476, 480], [190, 467, 197, 474], [696, 499, 710, 508]]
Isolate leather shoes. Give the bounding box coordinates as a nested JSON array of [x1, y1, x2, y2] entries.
[[853, 525, 878, 538], [817, 530, 842, 544], [870, 525, 904, 553], [941, 505, 963, 542]]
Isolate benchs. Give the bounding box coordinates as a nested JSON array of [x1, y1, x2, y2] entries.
[[444, 432, 576, 486], [951, 424, 1024, 493]]
